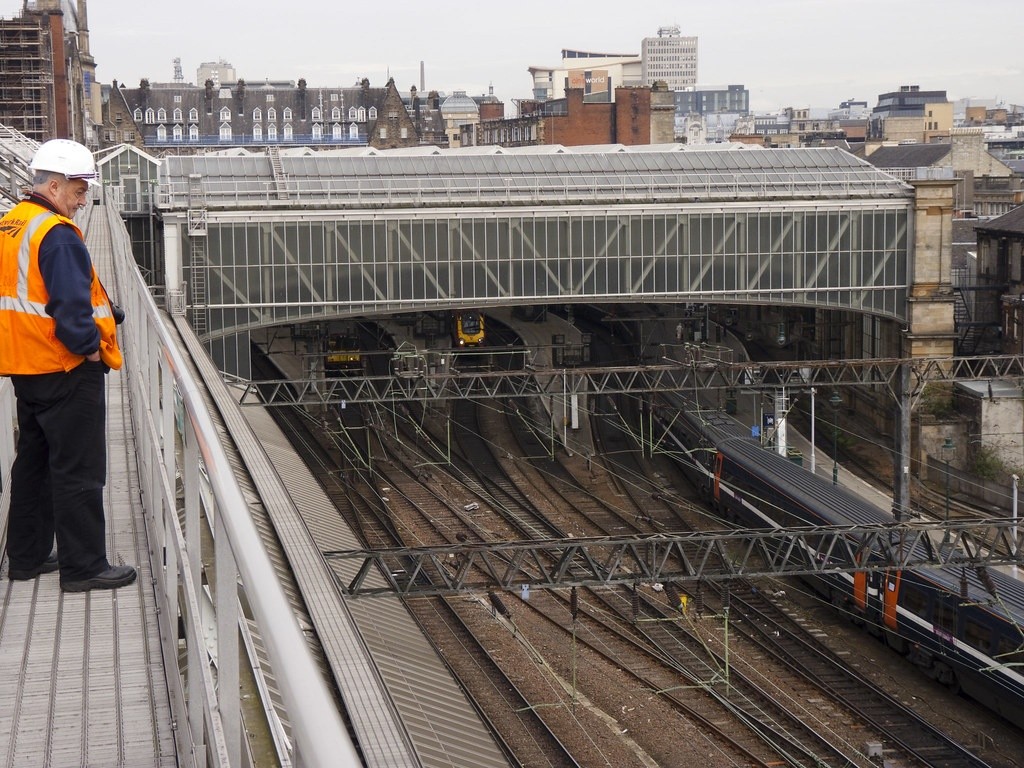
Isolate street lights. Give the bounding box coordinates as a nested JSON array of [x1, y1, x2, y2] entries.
[[829, 391, 843, 484], [942, 437, 957, 542]]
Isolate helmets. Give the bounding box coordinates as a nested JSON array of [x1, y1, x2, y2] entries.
[[28, 139, 95, 181]]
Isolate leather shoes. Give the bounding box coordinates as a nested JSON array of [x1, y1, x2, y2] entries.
[[8, 550, 59, 580], [59, 566, 137, 592]]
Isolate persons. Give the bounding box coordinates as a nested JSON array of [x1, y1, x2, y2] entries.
[[0, 138, 138, 592]]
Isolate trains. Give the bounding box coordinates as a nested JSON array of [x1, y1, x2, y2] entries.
[[450, 308, 484, 347], [323, 320, 363, 377], [586, 302, 1024, 732]]
